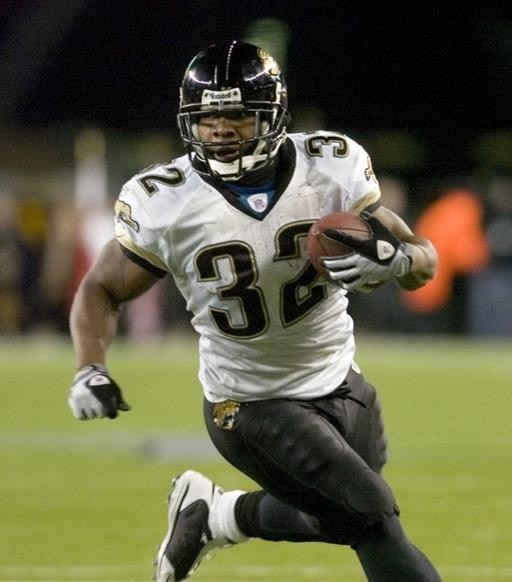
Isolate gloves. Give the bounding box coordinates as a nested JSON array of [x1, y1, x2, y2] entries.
[[67, 362, 132, 421], [318, 209, 413, 294]]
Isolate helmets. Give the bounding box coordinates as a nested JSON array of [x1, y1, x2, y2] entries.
[[176, 39, 290, 185]]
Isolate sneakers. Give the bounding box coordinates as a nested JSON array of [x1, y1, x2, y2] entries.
[[150, 468, 239, 582]]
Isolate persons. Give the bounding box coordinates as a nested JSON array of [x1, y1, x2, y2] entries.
[[68, 40, 442, 582]]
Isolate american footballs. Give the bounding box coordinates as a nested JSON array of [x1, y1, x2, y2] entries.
[[305, 213, 394, 288]]
